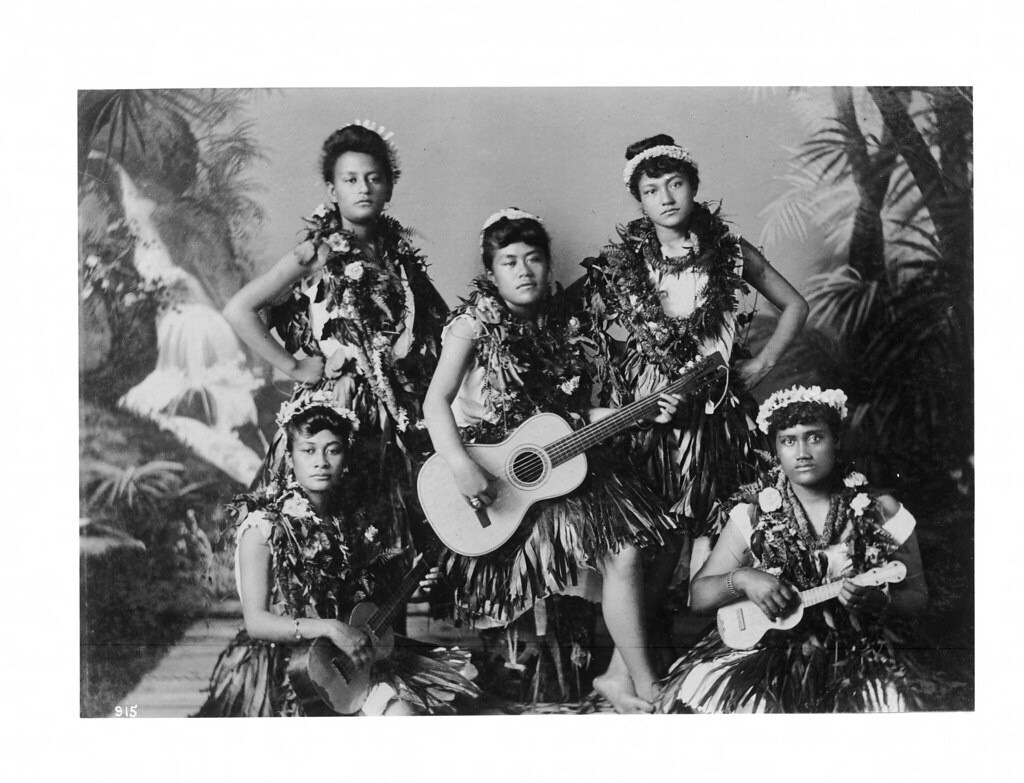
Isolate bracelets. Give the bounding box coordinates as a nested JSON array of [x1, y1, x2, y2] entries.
[[292, 618, 304, 640], [727, 568, 740, 599]]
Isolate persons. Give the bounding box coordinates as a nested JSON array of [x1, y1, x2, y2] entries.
[[653, 384, 937, 713], [186, 397, 483, 716], [421, 196, 672, 714], [222, 121, 450, 573], [562, 133, 810, 609]]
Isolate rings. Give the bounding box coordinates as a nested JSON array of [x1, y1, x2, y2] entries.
[[470, 499, 482, 508], [854, 604, 858, 608]]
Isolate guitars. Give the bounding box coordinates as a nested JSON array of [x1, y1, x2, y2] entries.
[[716, 559, 909, 651], [416, 350, 731, 558], [286, 556, 433, 717]]
